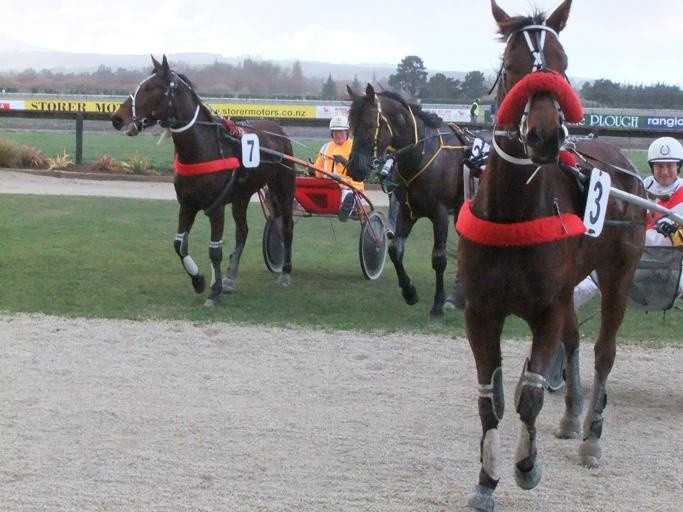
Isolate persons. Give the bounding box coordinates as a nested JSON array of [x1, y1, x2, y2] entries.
[[643, 137, 683, 246], [470, 98, 480, 123], [314, 115, 365, 219], [490, 96, 497, 124]]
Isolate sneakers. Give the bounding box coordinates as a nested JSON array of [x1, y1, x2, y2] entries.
[[338, 192, 355, 222]]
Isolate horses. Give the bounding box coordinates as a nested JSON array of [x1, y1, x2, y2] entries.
[[344, 82, 494, 329], [110, 53, 296, 310], [455, 0, 650, 512]]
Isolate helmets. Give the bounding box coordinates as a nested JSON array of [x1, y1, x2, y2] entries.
[[328, 116, 349, 130], [647, 136, 683, 174]]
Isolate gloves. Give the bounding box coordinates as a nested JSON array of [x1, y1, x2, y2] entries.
[[306, 158, 314, 176], [333, 155, 347, 165], [657, 222, 673, 237]]
